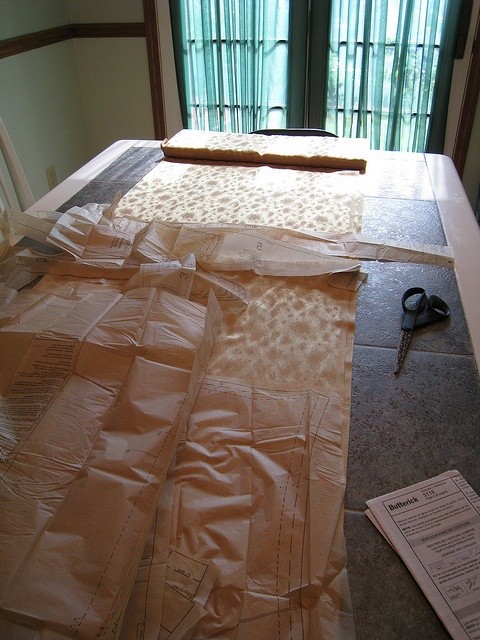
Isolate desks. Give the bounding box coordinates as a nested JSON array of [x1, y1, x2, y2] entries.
[[1, 137, 480, 639]]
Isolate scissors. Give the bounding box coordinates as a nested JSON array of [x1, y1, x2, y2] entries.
[[393, 287, 451, 374]]
